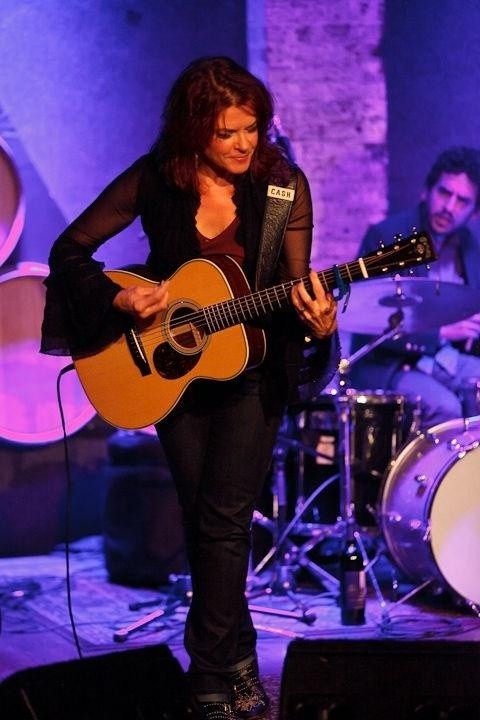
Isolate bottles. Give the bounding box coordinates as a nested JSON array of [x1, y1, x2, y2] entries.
[[340, 517, 366, 625]]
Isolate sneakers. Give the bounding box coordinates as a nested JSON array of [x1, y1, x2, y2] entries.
[[198, 657, 270, 720]]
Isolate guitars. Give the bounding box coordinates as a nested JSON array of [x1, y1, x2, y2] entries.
[[71, 226, 439, 429]]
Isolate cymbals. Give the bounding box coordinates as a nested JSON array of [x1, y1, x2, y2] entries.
[[336, 277, 480, 337]]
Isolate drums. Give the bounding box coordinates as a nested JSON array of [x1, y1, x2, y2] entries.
[[380, 414, 479, 613], [304, 386, 422, 501]]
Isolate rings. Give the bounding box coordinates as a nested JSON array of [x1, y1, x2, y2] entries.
[[298, 307, 305, 314]]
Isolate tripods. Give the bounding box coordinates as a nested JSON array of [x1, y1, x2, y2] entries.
[[276, 322, 391, 629], [109, 411, 318, 643]]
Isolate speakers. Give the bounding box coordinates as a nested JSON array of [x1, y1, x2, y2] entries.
[[274, 635, 479, 720], [0, 641, 208, 719]]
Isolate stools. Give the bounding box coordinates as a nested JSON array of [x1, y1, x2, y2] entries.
[[287, 389, 406, 622]]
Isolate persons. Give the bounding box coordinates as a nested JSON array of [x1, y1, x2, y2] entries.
[[36, 53, 344, 720], [334, 147, 480, 439]]
